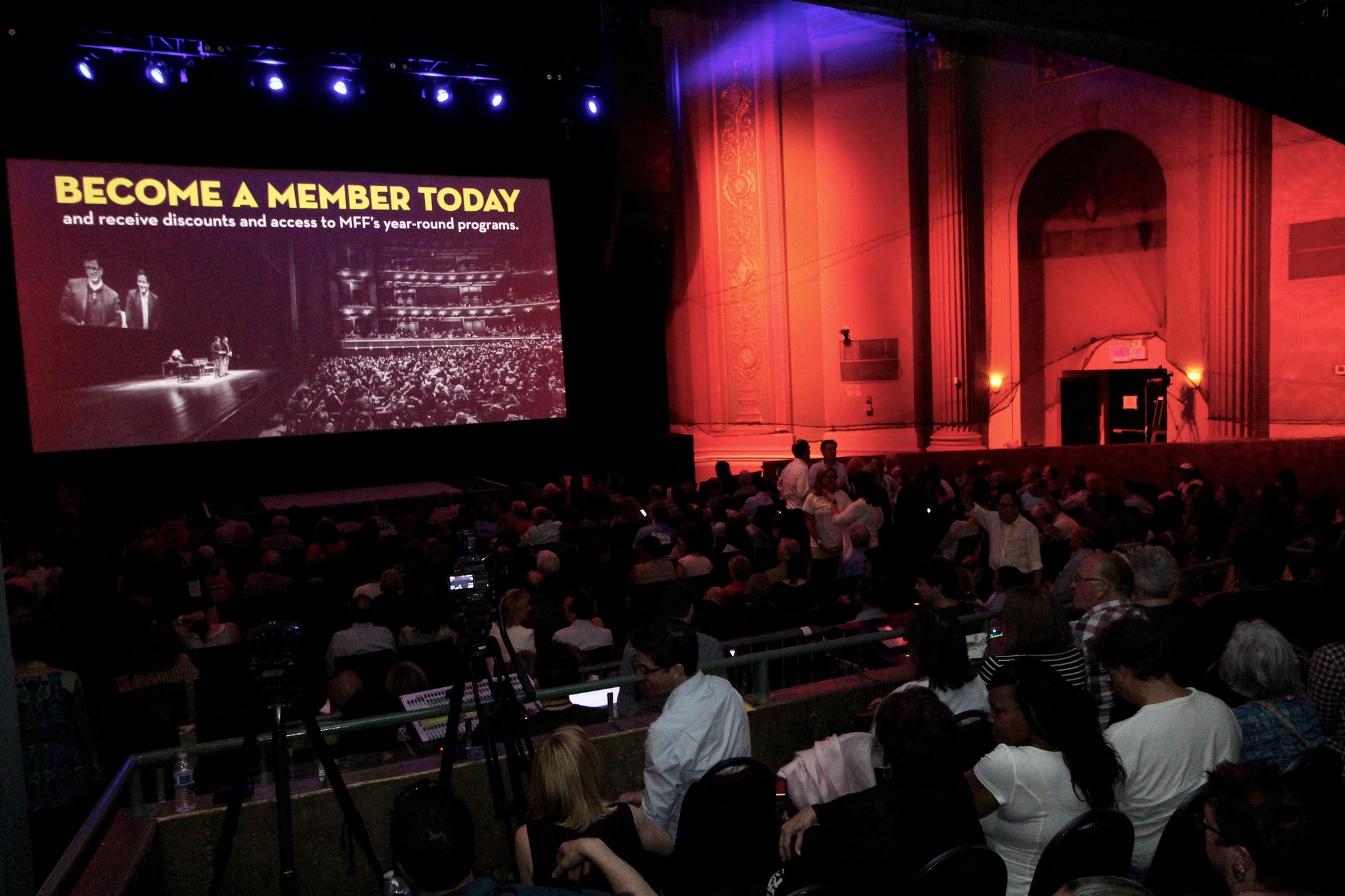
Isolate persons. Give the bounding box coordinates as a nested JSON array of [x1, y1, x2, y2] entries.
[[514, 723, 674, 893], [1049, 874, 1153, 896], [10, 621, 99, 896], [1091, 612, 1245, 883], [281, 250, 568, 434], [960, 652, 1128, 896], [57, 250, 123, 328], [614, 616, 753, 866], [211, 335, 227, 378], [221, 336, 232, 375], [124, 268, 166, 331], [0, 439, 1345, 764], [1200, 757, 1333, 896], [388, 775, 658, 896], [777, 685, 986, 896]]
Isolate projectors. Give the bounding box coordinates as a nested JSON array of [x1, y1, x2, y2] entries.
[[397, 671, 551, 756]]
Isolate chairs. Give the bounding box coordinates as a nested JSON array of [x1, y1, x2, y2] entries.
[[667, 699, 1345, 895]]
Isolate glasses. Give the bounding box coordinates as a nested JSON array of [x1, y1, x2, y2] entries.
[[1194, 812, 1225, 839], [1073, 574, 1105, 583], [676, 540, 686, 545], [630, 655, 665, 676], [996, 502, 1015, 509], [822, 474, 839, 480]]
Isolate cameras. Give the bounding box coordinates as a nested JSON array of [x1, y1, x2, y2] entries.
[[448, 530, 510, 608]]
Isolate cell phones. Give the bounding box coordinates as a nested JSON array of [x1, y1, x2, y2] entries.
[[639, 508, 648, 517]]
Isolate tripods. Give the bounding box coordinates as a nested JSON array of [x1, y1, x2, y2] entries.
[[211, 620, 388, 896], [438, 617, 539, 880]]
